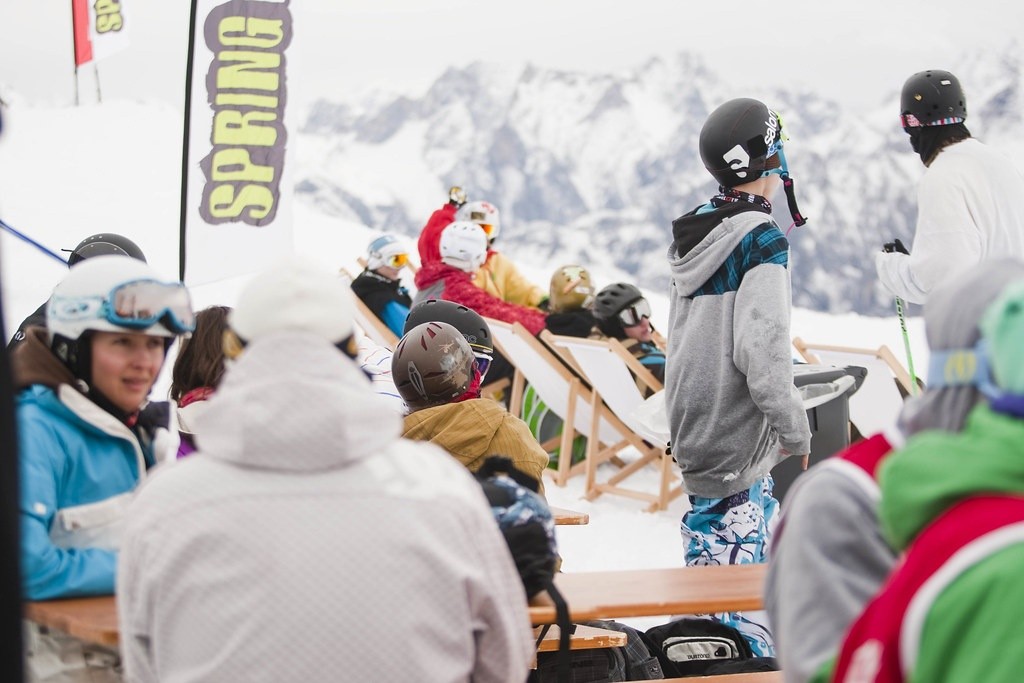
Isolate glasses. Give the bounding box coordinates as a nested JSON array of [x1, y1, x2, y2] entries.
[[617, 298, 650, 326], [108, 278, 196, 332], [473, 352, 494, 385], [478, 223, 495, 240], [390, 254, 408, 270], [777, 114, 790, 140]]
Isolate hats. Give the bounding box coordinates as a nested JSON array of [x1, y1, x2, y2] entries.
[[228, 267, 355, 344]]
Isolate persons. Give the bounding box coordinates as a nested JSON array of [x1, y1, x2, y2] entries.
[[0, 184, 1022, 682], [875, 69, 1024, 397], [663, 96, 813, 663], [114, 260, 536, 682], [1, 255, 197, 605]]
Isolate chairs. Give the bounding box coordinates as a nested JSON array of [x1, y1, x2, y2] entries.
[[338, 256, 683, 515], [792, 337, 926, 452]]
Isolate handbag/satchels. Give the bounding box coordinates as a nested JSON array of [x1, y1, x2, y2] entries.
[[527, 615, 778, 683]]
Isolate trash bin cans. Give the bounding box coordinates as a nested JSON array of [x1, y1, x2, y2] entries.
[[770, 364, 868, 512]]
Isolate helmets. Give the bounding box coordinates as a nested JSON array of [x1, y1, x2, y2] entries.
[[455, 201, 500, 239], [367, 235, 405, 270], [391, 322, 474, 412], [549, 266, 590, 308], [439, 221, 487, 265], [67, 233, 148, 266], [44, 254, 192, 348], [403, 299, 493, 356], [593, 282, 642, 340], [900, 70, 967, 125], [699, 98, 780, 187]]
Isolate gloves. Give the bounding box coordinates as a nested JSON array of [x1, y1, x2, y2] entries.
[[449, 187, 467, 205]]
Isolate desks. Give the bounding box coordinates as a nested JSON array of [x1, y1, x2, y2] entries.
[[23, 592, 119, 647], [524, 561, 782, 683]]
